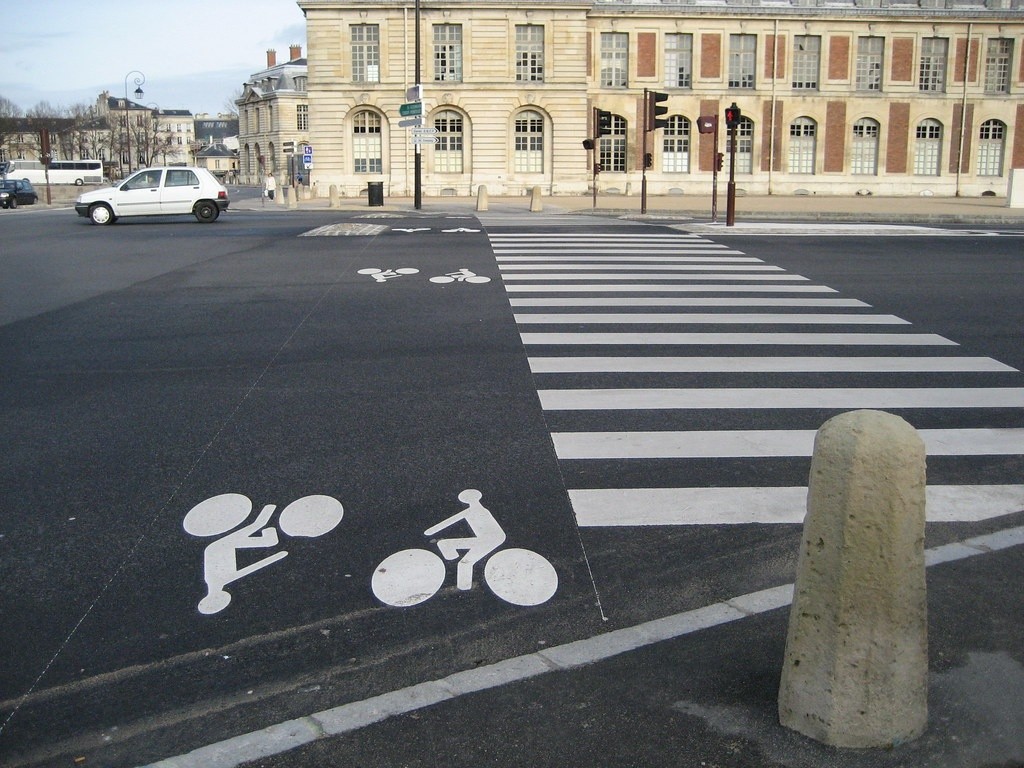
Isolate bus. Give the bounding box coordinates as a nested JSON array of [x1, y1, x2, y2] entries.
[[0, 159, 105, 187]]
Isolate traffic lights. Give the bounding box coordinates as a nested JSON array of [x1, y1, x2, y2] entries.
[[724, 107, 741, 126], [583, 139, 593, 149], [649, 92, 669, 131], [596, 109, 611, 138]]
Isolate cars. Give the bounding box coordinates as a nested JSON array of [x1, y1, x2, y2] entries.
[[0, 179, 39, 210]]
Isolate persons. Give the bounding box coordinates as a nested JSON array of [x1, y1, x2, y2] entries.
[[297, 172, 302, 184], [266, 173, 276, 201]]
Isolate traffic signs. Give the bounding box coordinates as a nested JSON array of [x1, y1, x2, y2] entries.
[[399, 101, 422, 117], [397, 117, 425, 128], [411, 136, 439, 145], [411, 127, 439, 135], [405, 84, 422, 102]]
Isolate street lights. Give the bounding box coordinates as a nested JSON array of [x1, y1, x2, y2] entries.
[[124, 69, 146, 176]]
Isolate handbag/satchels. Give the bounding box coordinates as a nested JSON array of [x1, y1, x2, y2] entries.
[[265, 190, 269, 197]]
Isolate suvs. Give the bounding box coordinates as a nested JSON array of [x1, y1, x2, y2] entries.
[[74, 164, 231, 227]]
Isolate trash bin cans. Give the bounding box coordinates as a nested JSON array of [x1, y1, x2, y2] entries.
[[367, 182, 383, 206]]
